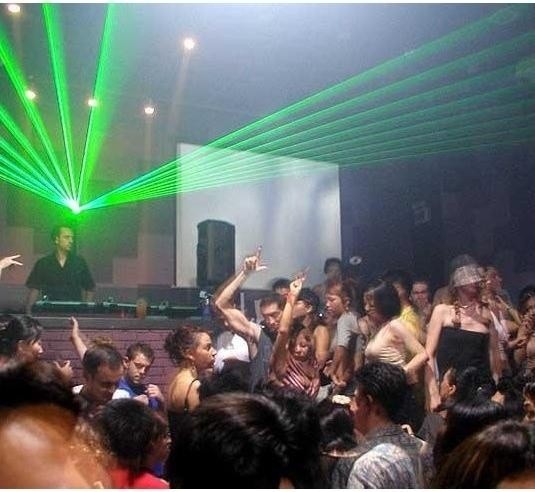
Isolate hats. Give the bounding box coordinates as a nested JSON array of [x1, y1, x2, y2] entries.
[[447, 254, 486, 292]]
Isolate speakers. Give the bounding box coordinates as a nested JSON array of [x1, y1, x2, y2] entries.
[[197, 219, 235, 288]]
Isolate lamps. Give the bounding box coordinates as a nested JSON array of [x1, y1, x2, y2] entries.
[[144, 97, 154, 115]]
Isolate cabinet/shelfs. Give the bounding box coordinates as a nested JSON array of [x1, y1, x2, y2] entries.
[[36, 316, 204, 395]]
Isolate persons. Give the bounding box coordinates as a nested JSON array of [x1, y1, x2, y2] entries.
[[70, 347, 123, 422], [172, 392, 295, 488], [439, 422, 533, 490], [411, 279, 432, 321], [425, 363, 484, 443], [485, 266, 524, 329], [363, 280, 428, 427], [270, 279, 321, 403], [165, 323, 216, 486], [512, 286, 535, 368], [0, 317, 74, 399], [290, 288, 328, 368], [295, 400, 357, 490], [312, 259, 352, 306], [268, 326, 319, 402], [346, 363, 435, 488], [380, 269, 426, 410], [430, 401, 503, 455], [214, 245, 286, 395], [196, 368, 241, 406], [87, 399, 170, 488], [2, 255, 24, 339], [427, 264, 500, 414], [490, 366, 533, 431], [72, 316, 165, 479], [270, 277, 288, 302], [2, 363, 110, 488], [24, 226, 95, 322], [323, 281, 359, 394]]
